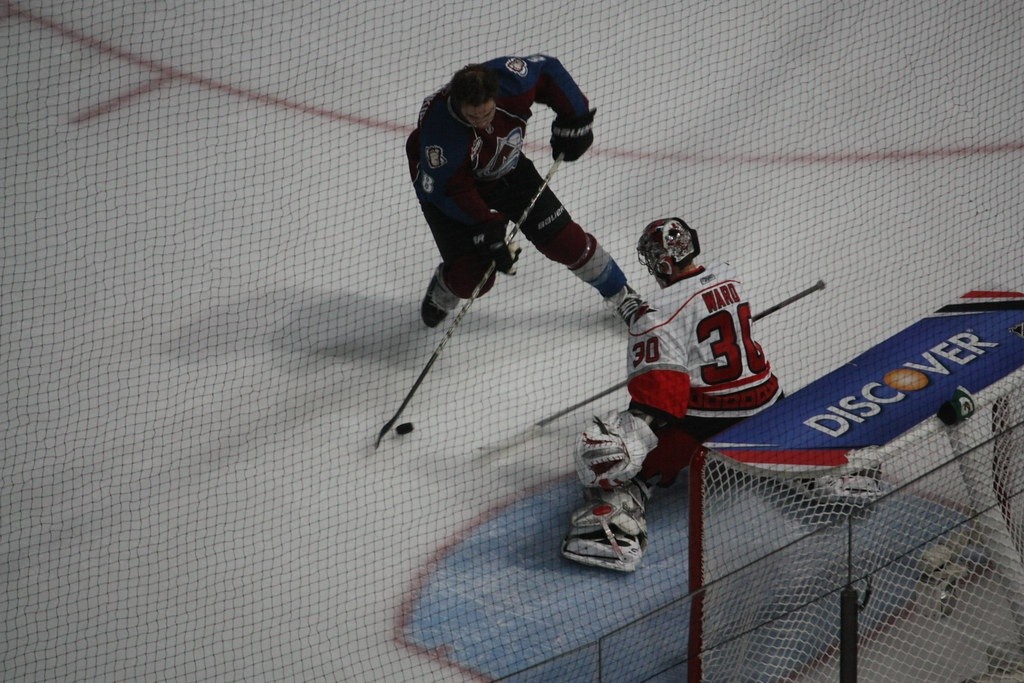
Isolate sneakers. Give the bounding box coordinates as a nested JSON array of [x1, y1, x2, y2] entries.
[[601, 284, 641, 327], [566, 529, 640, 559], [420, 263, 461, 330]]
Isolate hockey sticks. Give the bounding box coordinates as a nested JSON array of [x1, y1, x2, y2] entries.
[[374, 104, 599, 450], [475, 279, 828, 452]]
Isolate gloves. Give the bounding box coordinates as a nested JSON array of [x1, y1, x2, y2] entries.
[[551, 108, 596, 162], [472, 222, 523, 276]]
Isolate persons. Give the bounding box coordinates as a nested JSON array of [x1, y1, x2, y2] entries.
[[561, 217, 882, 573], [405, 53, 650, 328]]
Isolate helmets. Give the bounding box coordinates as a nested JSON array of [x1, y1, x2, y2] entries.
[[637, 217, 701, 274]]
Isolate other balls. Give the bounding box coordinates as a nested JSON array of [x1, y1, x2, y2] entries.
[[396, 422, 413, 435]]
